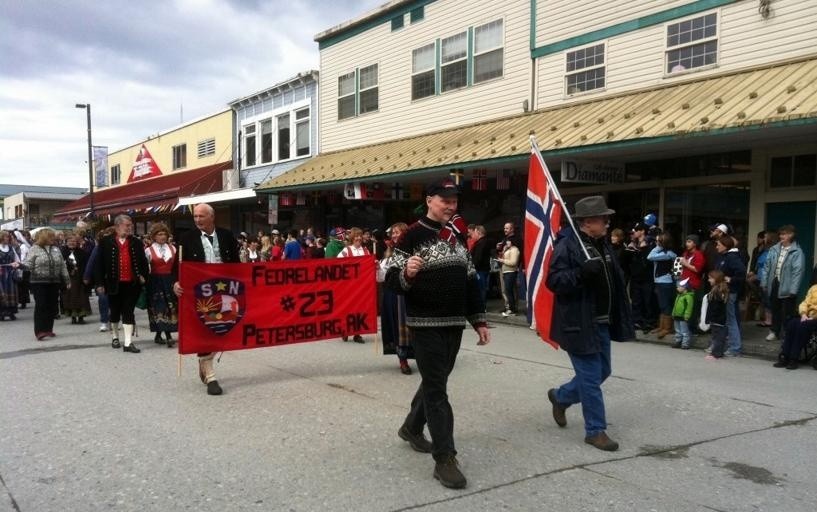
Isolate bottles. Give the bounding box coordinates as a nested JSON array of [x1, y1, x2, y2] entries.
[[569, 196, 615, 218], [426, 177, 461, 196]]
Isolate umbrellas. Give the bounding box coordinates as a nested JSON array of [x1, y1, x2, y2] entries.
[[401, 366, 411, 375], [207, 380, 221, 395], [99, 323, 176, 353]]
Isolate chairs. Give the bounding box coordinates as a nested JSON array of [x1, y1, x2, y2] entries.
[[548, 389, 570, 426], [434, 453, 467, 489], [354, 335, 365, 343], [585, 431, 618, 451], [398, 423, 432, 452]]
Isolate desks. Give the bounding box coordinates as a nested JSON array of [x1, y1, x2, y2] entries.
[[74, 103, 94, 222]]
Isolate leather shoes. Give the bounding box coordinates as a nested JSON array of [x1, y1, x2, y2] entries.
[[586, 260, 602, 276]]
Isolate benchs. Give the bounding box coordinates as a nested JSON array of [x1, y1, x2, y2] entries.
[[524, 147, 562, 352]]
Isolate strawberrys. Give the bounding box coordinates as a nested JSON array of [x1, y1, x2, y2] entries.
[[776, 316, 817, 372]]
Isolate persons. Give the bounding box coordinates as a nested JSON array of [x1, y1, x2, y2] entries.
[[384, 177, 493, 490], [609, 211, 817, 370], [172, 202, 240, 395], [235, 219, 418, 375], [462, 220, 525, 317], [0, 213, 187, 354], [544, 196, 620, 451]]
